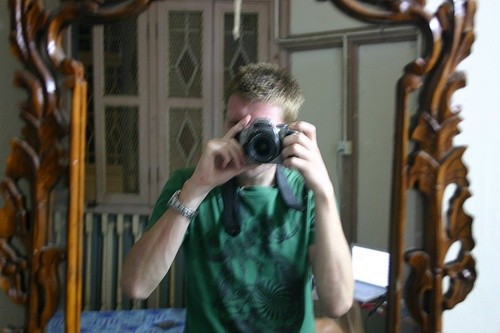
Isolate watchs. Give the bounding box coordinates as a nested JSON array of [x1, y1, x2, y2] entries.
[[167, 190, 198, 221]]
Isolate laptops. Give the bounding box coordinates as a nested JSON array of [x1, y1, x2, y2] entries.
[[349, 242, 390, 303]]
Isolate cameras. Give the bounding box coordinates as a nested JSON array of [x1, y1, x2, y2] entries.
[[235, 118, 298, 164]]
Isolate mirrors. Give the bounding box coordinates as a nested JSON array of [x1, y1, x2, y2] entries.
[[0, 0, 478, 332]]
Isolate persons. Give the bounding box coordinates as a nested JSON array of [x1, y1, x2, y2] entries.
[[120, 63, 354, 333]]
